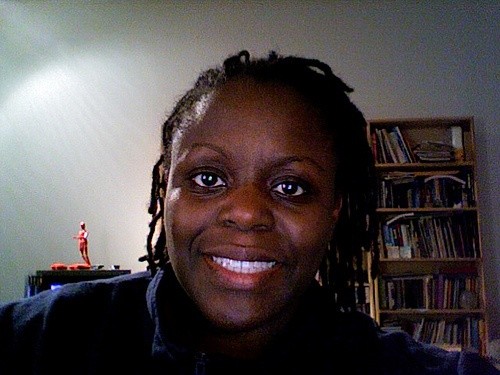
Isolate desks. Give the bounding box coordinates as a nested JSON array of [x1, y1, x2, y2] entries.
[[36, 270, 131, 297]]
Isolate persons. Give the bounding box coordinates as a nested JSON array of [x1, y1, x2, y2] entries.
[[72, 222, 91, 265], [1, 52, 500, 374]]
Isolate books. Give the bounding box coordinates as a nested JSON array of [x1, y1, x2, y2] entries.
[[380, 312, 485, 357], [351, 217, 370, 317], [370, 122, 468, 163], [379, 170, 477, 208], [375, 213, 479, 260], [378, 268, 483, 310]]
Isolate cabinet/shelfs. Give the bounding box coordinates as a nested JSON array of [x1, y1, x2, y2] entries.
[[318, 117, 488, 357]]
